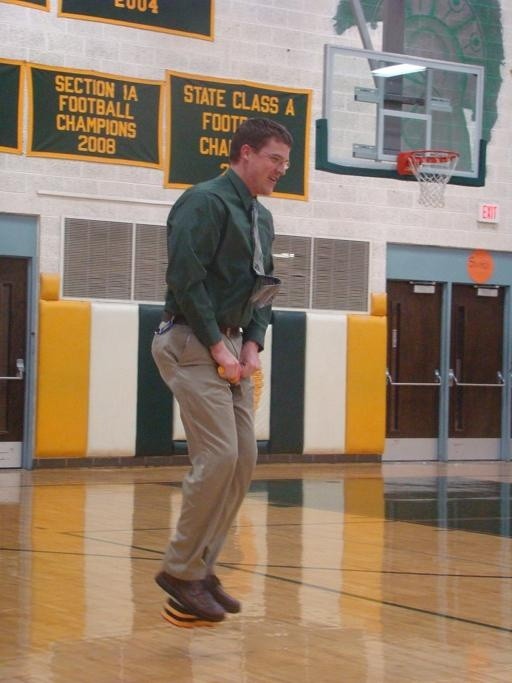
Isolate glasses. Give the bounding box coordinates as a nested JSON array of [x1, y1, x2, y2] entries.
[[261, 151, 290, 169]]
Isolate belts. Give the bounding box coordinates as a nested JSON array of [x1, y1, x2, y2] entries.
[[162, 311, 239, 337]]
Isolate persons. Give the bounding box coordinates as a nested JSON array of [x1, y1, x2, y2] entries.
[[149, 119, 294, 624]]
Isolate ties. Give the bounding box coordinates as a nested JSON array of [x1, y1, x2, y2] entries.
[[251, 198, 265, 275]]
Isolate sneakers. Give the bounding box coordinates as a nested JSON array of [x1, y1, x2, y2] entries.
[[155, 571, 242, 621]]
